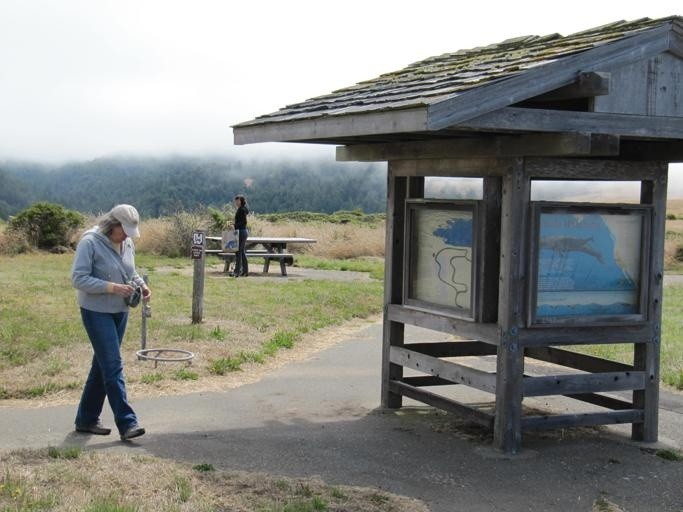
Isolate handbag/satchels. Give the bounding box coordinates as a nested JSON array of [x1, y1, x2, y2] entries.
[[221, 230, 240, 253]]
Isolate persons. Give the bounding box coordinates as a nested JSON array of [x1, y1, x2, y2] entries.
[[68, 203, 152, 442], [228, 195, 249, 278]]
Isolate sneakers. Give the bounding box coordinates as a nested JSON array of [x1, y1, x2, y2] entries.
[[120, 427, 145, 440], [76, 425, 111, 435]]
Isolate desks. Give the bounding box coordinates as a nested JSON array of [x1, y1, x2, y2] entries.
[[204, 235, 316, 276]]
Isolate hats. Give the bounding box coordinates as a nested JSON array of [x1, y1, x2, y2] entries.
[[112, 205, 140, 238]]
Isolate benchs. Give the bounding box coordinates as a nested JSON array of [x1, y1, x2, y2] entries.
[[204, 249, 293, 276]]
